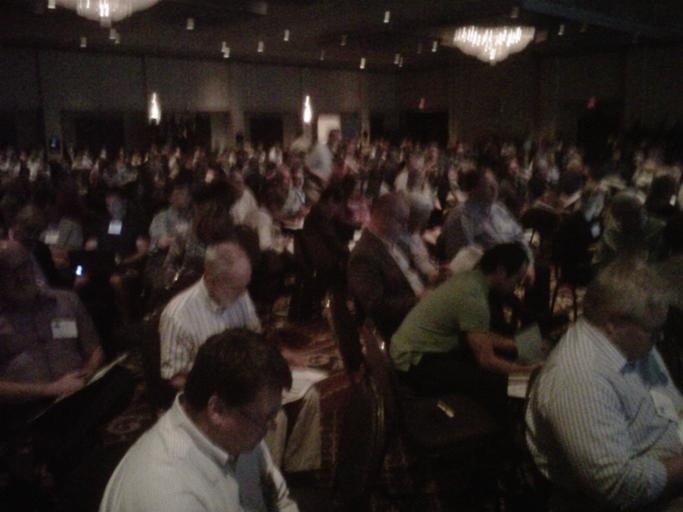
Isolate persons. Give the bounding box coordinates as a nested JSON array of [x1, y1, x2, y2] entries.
[[0, 128, 683, 512]]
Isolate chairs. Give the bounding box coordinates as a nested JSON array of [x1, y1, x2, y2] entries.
[[0, 198, 681, 512]]
